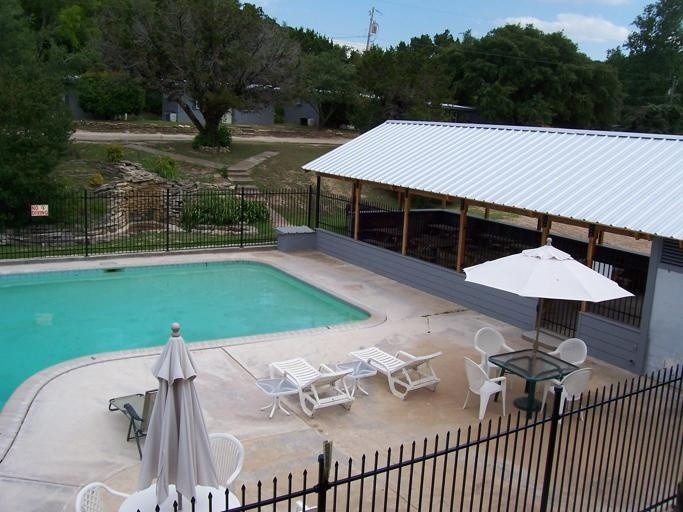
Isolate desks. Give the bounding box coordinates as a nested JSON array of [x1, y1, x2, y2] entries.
[[256, 378, 298, 418], [118, 484, 241, 512], [337, 362, 377, 398], [489, 349, 577, 411]]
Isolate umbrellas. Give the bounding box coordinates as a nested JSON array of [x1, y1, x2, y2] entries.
[[134, 323, 221, 511], [460, 236, 637, 375]]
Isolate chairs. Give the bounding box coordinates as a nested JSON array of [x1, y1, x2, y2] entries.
[[76, 482, 130, 512], [475, 327, 514, 378], [208, 434, 244, 490], [540, 368, 592, 425], [349, 347, 441, 400], [108, 389, 159, 460], [269, 359, 355, 419], [462, 356, 506, 420], [542, 338, 587, 379]]
